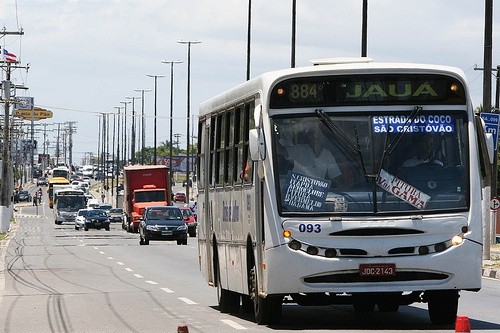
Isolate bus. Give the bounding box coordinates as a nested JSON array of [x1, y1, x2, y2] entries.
[[47, 166, 69, 209], [196, 56, 493, 325]]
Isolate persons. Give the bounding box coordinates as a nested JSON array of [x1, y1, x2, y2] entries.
[[240, 126, 444, 190], [101, 192, 105, 202], [32, 189, 42, 206], [168, 210, 175, 218]]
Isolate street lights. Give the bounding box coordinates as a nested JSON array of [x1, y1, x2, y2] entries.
[[147, 75, 166, 164], [178, 41, 201, 203], [135, 90, 150, 164], [163, 61, 183, 179], [97, 96, 142, 196]]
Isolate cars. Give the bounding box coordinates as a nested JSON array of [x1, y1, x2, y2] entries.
[[74, 203, 122, 231], [139, 207, 188, 246], [70, 165, 117, 190], [20, 191, 32, 202], [178, 207, 197, 237], [33, 169, 49, 187], [183, 178, 192, 187]]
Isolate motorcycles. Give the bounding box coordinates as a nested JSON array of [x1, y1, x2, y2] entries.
[[39, 191, 42, 196]]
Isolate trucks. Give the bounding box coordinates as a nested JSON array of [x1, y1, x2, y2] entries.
[[52, 185, 88, 225], [122, 164, 170, 232]]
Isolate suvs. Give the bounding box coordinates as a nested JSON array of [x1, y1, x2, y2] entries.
[[175, 193, 186, 202]]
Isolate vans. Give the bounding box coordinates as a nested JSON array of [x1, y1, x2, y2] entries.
[[82, 165, 94, 178], [86, 199, 99, 209]]
